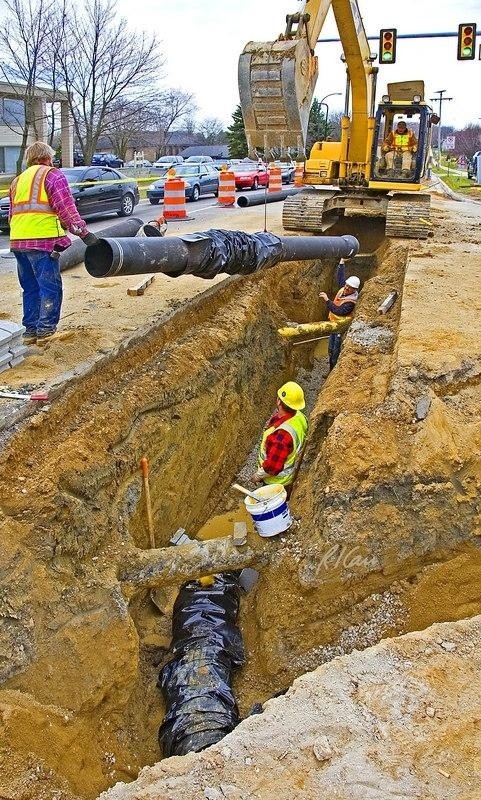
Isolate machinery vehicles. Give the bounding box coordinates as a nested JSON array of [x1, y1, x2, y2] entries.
[[237, 0, 438, 239]]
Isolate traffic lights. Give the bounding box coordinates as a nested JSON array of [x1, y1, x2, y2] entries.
[[457, 23, 476, 60], [379, 27, 397, 64]]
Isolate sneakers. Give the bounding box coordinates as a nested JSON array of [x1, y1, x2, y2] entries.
[[37, 331, 75, 346], [22, 338, 37, 345]]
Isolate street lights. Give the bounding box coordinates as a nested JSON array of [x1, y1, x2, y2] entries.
[[316, 92, 344, 142]]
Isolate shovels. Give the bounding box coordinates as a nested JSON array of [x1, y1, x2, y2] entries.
[[140, 458, 180, 617]]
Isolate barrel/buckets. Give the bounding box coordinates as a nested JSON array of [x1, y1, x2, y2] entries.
[[242, 484, 294, 538]]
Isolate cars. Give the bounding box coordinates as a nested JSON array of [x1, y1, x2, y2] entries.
[[49, 149, 299, 185], [0, 166, 140, 231], [147, 162, 221, 205], [228, 163, 270, 190], [467, 150, 481, 180]]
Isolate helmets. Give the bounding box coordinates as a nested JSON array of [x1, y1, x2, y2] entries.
[[278, 381, 306, 411], [345, 276, 360, 289]]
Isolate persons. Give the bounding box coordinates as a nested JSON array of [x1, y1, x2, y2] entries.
[[9, 140, 100, 346], [319, 258, 360, 379], [381, 121, 418, 178], [250, 381, 308, 501]]
